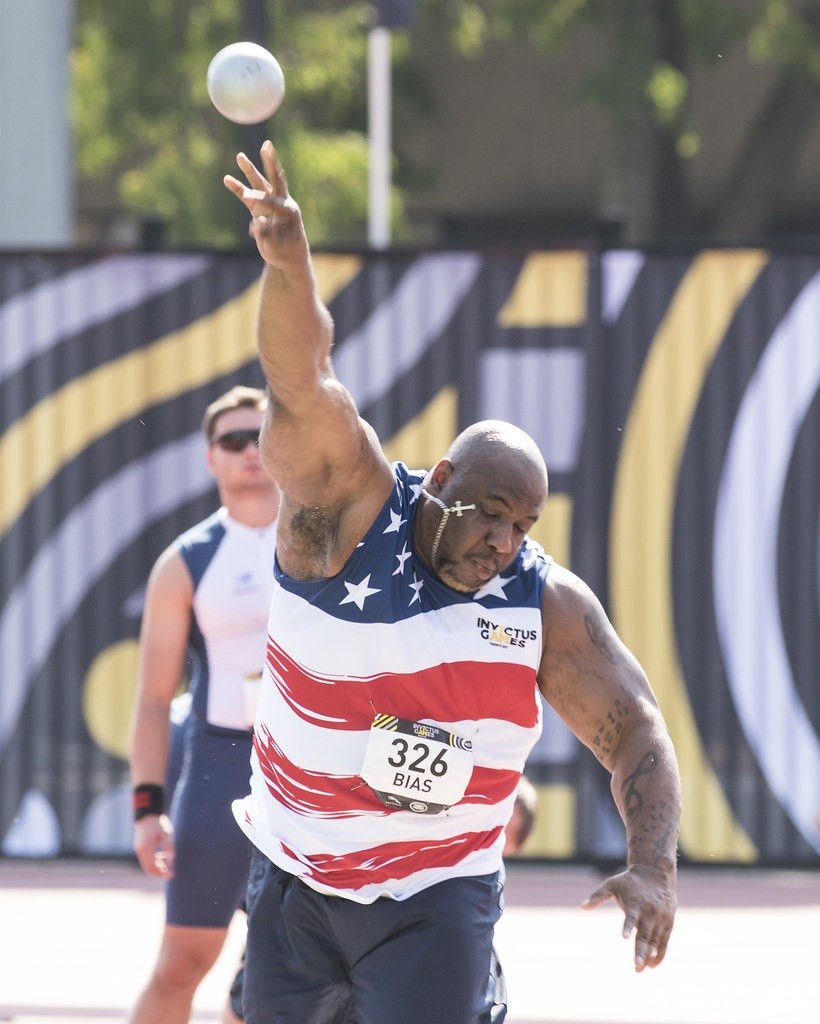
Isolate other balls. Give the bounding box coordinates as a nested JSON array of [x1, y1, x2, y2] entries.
[[206, 38, 289, 125]]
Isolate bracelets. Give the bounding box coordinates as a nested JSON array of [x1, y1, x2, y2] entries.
[[133, 783, 169, 819]]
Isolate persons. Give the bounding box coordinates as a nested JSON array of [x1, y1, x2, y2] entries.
[[223, 134, 682, 1024], [134, 388, 538, 1023]]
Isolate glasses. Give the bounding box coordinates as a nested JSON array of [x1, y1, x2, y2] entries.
[[212, 430, 259, 451]]
